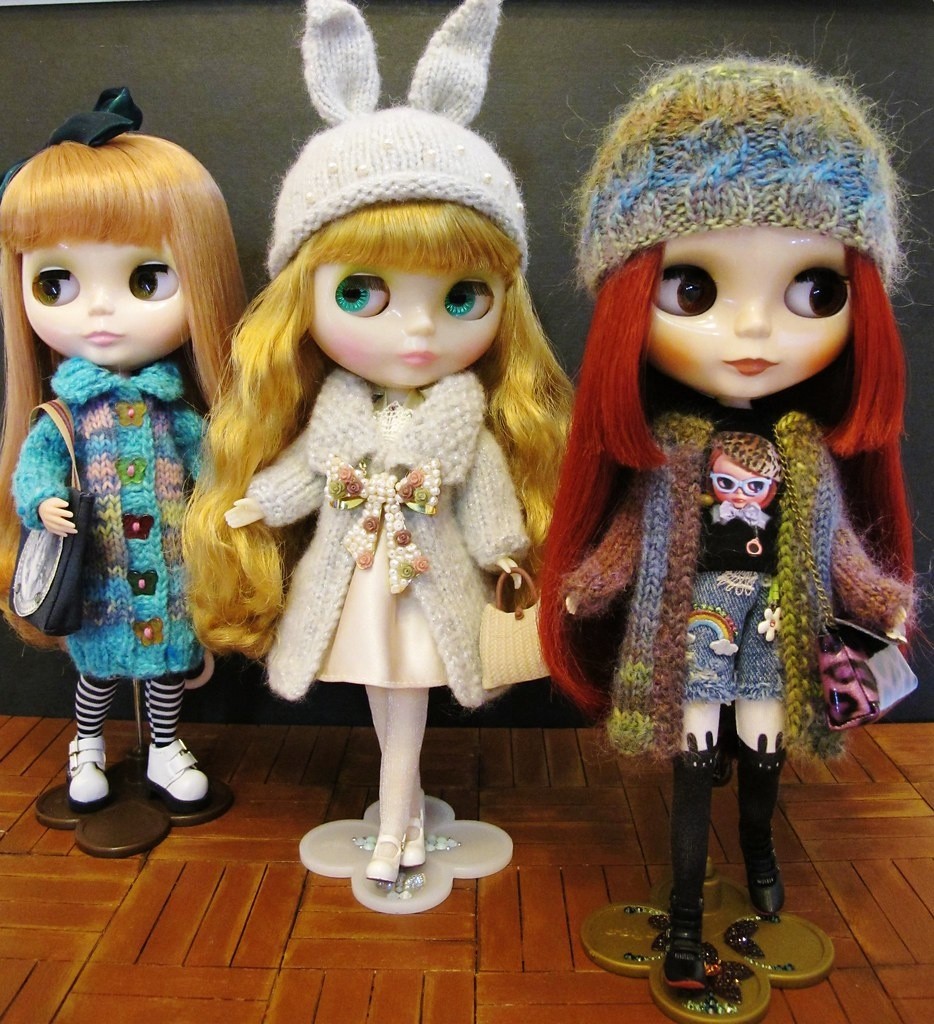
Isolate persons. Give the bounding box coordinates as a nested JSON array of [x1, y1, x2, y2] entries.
[[181, 0, 573, 884], [538, 59, 916, 990], [0, 86, 247, 813]]
